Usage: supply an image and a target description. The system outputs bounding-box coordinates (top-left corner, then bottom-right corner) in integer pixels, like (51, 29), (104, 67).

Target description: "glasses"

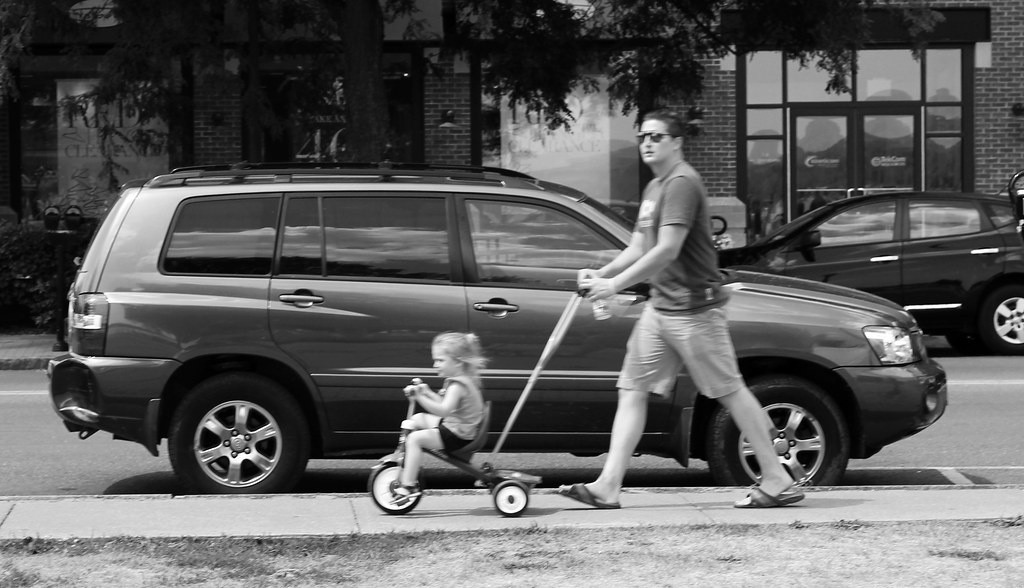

(636, 131), (680, 143)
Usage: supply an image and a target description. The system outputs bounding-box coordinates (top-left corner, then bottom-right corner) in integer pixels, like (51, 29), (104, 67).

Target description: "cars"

(722, 192), (1024, 357)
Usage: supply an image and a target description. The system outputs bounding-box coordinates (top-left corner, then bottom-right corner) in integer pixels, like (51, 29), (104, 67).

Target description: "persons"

(388, 331), (486, 505)
(551, 111), (805, 509)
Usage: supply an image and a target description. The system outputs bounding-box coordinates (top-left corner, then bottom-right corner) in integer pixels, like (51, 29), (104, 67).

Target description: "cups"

(592, 298), (613, 319)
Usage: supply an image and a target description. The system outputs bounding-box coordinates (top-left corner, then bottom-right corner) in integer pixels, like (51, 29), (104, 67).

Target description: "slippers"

(553, 482), (621, 509)
(734, 487), (805, 508)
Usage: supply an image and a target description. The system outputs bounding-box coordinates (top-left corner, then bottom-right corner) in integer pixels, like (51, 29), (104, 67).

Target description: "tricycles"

(368, 375), (544, 515)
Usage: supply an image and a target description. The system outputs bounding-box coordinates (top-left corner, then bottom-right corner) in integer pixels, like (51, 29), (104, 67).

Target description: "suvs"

(48, 162), (947, 496)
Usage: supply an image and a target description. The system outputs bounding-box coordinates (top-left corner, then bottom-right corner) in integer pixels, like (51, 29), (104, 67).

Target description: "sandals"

(389, 481), (422, 505)
